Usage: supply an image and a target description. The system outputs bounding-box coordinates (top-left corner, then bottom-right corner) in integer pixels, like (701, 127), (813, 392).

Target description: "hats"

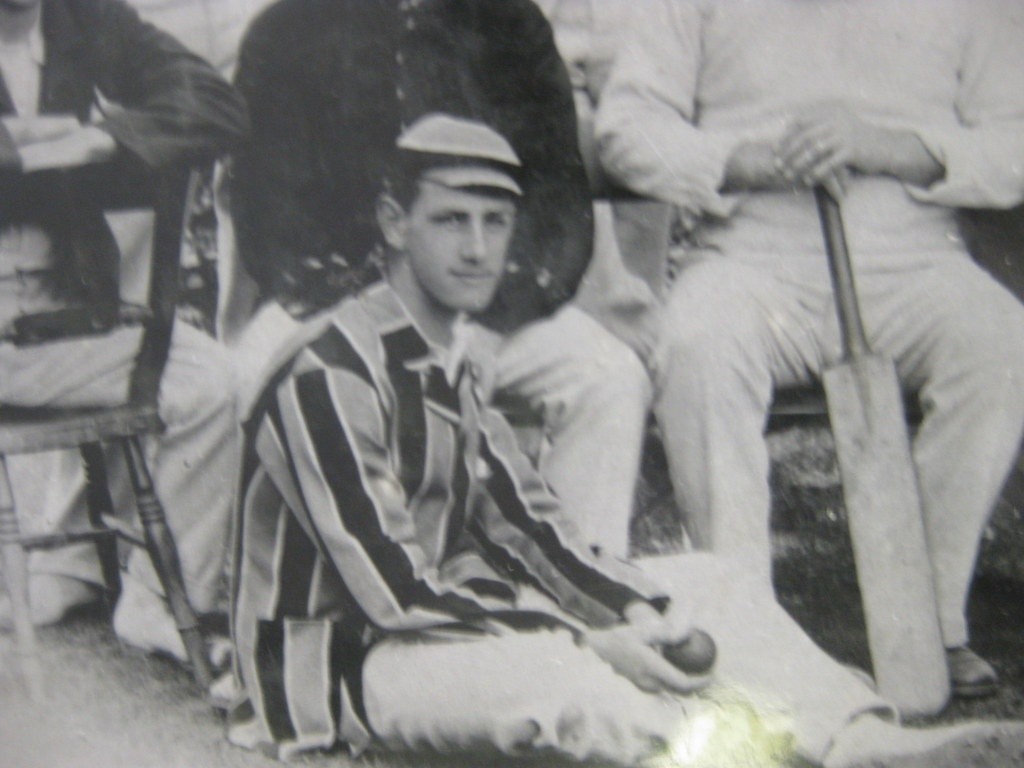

(396, 110), (523, 201)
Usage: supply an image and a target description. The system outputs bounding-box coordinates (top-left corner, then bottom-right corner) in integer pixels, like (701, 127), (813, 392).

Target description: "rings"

(817, 142), (828, 154)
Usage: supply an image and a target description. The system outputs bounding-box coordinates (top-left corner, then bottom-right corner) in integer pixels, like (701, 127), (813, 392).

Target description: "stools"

(0, 395), (208, 721)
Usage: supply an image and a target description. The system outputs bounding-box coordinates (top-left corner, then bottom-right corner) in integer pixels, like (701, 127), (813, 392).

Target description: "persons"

(0, 0), (251, 680)
(592, 0), (1024, 694)
(218, 0), (658, 557)
(225, 114), (1023, 768)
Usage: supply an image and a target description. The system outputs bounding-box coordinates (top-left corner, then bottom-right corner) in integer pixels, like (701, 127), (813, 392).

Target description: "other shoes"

(945, 645), (1002, 696)
(111, 586), (235, 670)
(823, 717), (1024, 768)
